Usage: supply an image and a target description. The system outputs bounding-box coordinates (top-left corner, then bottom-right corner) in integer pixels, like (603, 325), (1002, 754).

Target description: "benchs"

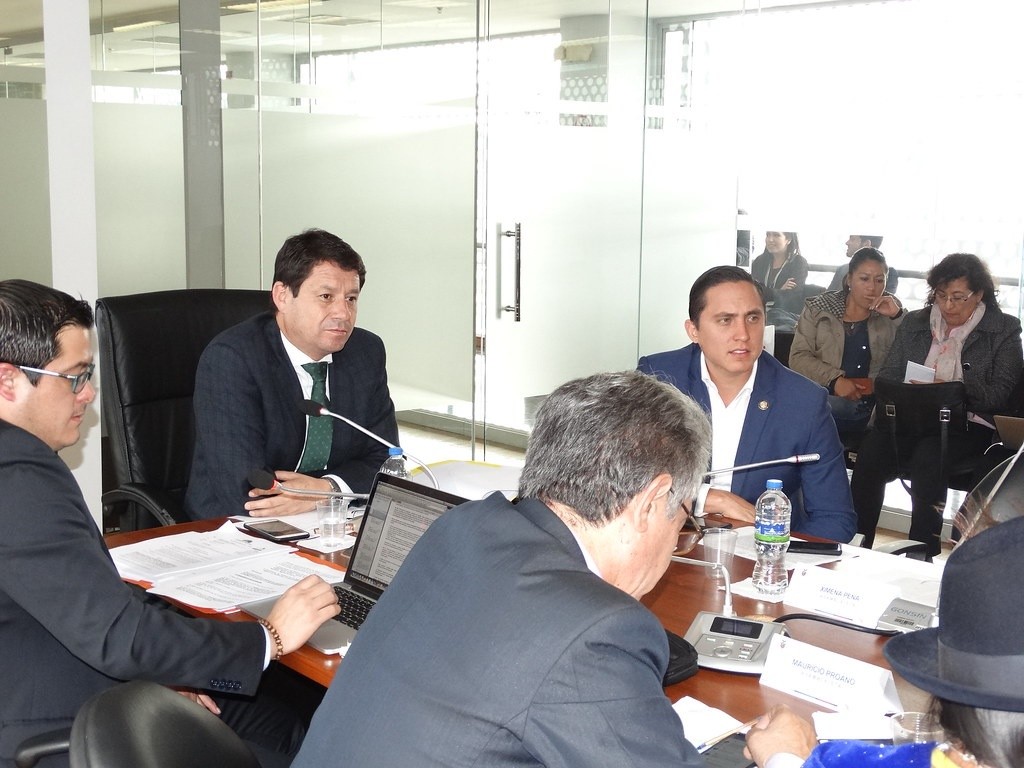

(773, 331), (1024, 491)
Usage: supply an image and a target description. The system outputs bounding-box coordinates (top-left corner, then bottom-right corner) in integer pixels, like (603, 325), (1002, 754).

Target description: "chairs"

(69, 681), (259, 768)
(798, 283), (828, 312)
(869, 445), (1023, 563)
(95, 288), (275, 536)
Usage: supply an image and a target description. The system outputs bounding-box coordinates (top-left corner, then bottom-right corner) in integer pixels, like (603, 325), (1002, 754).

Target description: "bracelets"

(257, 618), (284, 660)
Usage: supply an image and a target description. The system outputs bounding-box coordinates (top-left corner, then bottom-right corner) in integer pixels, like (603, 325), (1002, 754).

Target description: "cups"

(702, 527), (738, 580)
(315, 498), (350, 547)
(890, 711), (946, 746)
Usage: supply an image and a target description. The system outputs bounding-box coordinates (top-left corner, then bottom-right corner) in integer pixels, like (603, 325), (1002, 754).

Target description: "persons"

(850, 254), (1024, 563)
(787, 245), (909, 466)
(0, 278), (341, 768)
(180, 226), (406, 522)
(287, 364), (819, 767)
(826, 235), (897, 295)
(751, 231), (810, 308)
(635, 266), (859, 544)
(801, 515), (1024, 768)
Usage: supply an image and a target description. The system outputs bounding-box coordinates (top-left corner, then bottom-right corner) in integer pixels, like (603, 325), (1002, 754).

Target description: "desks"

(100, 512), (950, 768)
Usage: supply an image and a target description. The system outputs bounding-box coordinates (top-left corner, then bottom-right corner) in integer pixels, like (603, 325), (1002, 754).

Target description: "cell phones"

(698, 731), (758, 768)
(244, 519), (310, 541)
(683, 516), (733, 532)
(787, 541), (842, 556)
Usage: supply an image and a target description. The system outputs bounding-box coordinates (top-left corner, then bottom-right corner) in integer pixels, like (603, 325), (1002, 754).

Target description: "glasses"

(15, 364), (95, 395)
(933, 288), (974, 305)
(668, 488), (705, 555)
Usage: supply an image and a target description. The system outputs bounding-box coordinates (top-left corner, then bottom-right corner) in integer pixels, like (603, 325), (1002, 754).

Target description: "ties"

(296, 362), (333, 474)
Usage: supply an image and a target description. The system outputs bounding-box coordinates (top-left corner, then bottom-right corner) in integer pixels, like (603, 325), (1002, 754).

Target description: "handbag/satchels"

(875, 374), (966, 503)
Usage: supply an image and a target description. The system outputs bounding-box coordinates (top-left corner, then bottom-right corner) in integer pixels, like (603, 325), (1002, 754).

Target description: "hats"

(882, 515), (1024, 713)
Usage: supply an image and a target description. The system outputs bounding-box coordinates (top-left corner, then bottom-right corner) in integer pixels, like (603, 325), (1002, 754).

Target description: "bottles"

(752, 479), (792, 596)
(380, 447), (411, 483)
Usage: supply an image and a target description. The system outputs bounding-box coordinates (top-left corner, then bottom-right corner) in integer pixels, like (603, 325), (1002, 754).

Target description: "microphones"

(706, 453), (821, 476)
(296, 400), (439, 490)
(247, 470), (369, 499)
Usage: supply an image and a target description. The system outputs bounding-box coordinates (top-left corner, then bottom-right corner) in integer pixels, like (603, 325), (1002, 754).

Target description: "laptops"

(236, 472), (472, 654)
(993, 415), (1024, 450)
